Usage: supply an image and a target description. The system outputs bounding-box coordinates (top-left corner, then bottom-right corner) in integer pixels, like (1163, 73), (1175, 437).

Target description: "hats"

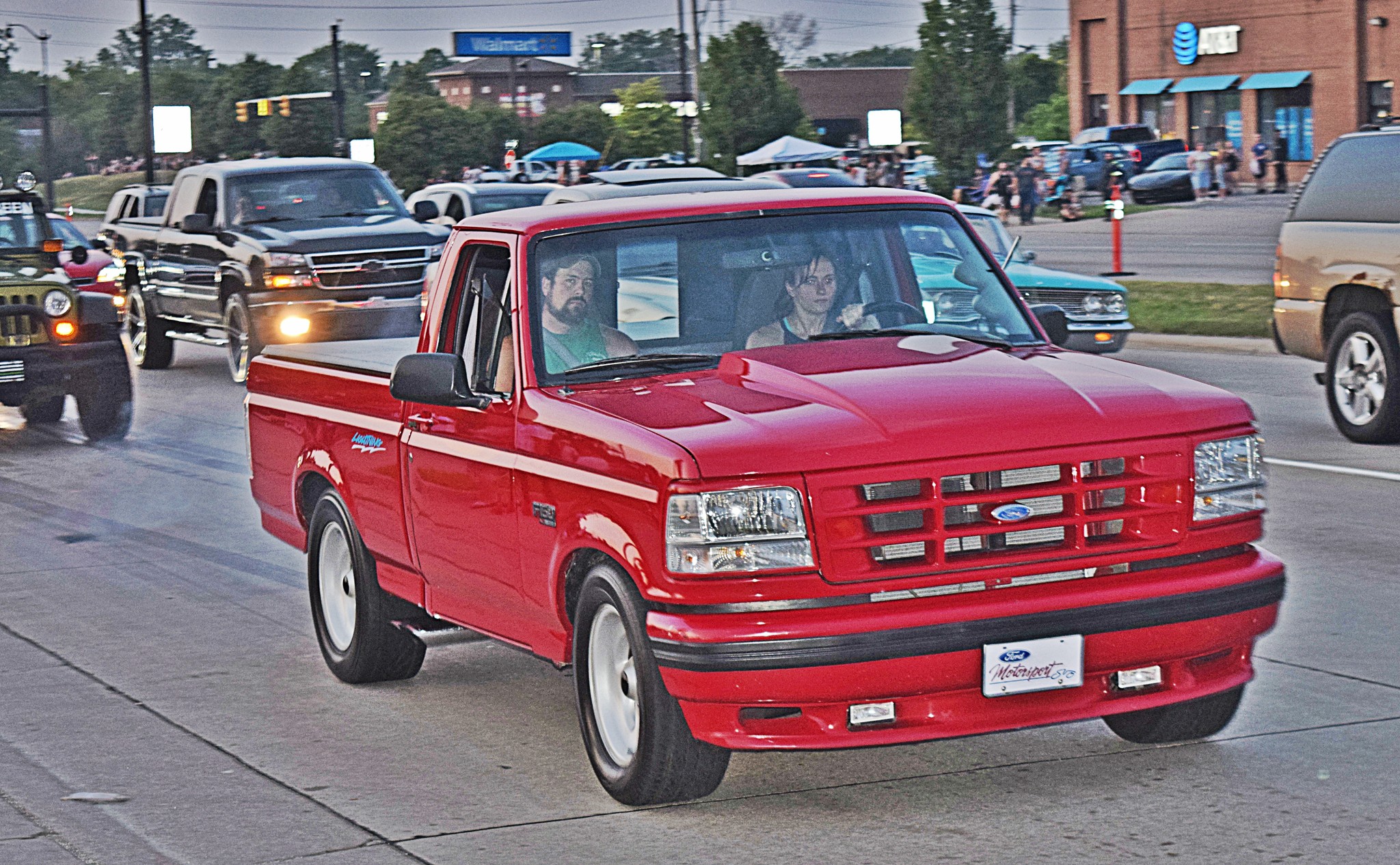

(539, 253), (602, 278)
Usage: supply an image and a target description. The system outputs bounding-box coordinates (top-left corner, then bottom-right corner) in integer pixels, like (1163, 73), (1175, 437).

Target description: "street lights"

(666, 34), (698, 166)
(2, 21), (55, 212)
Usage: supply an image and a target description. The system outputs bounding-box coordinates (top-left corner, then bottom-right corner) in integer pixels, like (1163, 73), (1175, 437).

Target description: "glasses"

(236, 201), (253, 206)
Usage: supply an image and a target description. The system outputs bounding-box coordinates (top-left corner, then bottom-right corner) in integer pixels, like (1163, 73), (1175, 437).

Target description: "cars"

(280, 158), (1134, 352)
(1128, 152), (1215, 205)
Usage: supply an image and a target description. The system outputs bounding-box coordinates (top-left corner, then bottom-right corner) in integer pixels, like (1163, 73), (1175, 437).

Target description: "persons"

(953, 145), (1084, 225)
(232, 191), (262, 225)
(1270, 129), (1290, 193)
(1250, 130), (1269, 194)
(1100, 152), (1123, 222)
(745, 246), (880, 352)
(849, 150), (932, 192)
(494, 253), (638, 396)
(1187, 140), (1240, 202)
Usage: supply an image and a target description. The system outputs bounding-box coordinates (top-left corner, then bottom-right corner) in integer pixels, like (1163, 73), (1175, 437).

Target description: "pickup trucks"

(241, 189), (1288, 809)
(1073, 126), (1186, 176)
(100, 156), (453, 385)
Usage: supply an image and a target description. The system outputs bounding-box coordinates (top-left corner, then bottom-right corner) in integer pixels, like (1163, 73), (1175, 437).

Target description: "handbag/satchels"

(1249, 158), (1261, 176)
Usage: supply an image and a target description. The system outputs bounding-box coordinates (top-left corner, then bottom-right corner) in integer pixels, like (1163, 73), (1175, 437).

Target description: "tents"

(735, 135), (844, 176)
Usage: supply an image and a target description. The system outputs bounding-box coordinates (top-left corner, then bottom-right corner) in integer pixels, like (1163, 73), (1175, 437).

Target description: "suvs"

(1036, 143), (1132, 191)
(1271, 114), (1400, 445)
(0, 172), (216, 444)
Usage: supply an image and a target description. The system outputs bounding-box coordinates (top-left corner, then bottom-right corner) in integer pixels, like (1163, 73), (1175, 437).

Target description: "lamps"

(1368, 18), (1385, 26)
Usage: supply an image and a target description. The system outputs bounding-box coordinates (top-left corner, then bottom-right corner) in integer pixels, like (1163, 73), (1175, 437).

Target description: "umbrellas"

(522, 142), (601, 161)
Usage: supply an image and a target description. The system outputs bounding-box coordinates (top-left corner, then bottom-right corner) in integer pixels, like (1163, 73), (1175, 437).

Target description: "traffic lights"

(236, 101), (247, 123)
(277, 97), (291, 118)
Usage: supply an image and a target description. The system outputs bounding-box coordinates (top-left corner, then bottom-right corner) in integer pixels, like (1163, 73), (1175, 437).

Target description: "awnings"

(1236, 71), (1313, 90)
(1118, 78), (1174, 95)
(1168, 75), (1237, 94)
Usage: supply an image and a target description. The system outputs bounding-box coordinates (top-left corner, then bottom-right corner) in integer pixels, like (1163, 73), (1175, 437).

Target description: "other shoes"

(1270, 190), (1286, 195)
(1195, 196), (1211, 202)
(1254, 188), (1267, 195)
